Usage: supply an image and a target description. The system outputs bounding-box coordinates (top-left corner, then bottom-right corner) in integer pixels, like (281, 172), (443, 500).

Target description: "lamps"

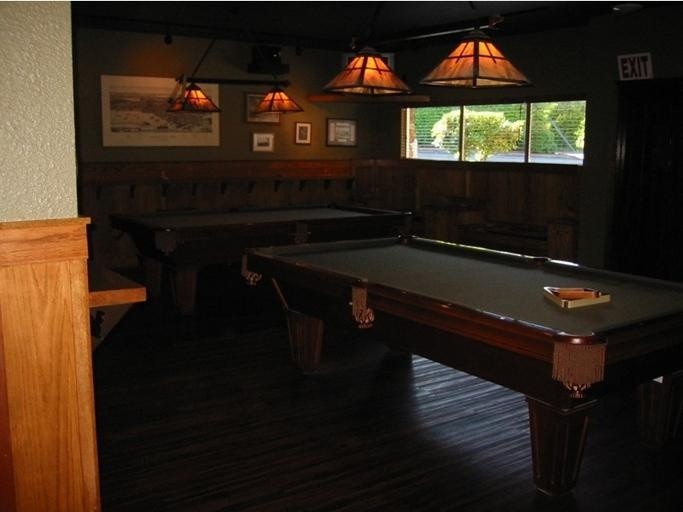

(321, 2), (415, 97)
(256, 72), (304, 114)
(418, 1), (533, 89)
(166, 37), (221, 112)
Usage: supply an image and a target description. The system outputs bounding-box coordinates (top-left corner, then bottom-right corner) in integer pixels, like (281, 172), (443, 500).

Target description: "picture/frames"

(244, 91), (281, 124)
(295, 122), (312, 145)
(100, 75), (221, 148)
(252, 133), (276, 154)
(326, 117), (359, 148)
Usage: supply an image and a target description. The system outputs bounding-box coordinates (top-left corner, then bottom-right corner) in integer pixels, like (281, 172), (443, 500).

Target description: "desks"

(108, 203), (414, 315)
(242, 233), (683, 498)
